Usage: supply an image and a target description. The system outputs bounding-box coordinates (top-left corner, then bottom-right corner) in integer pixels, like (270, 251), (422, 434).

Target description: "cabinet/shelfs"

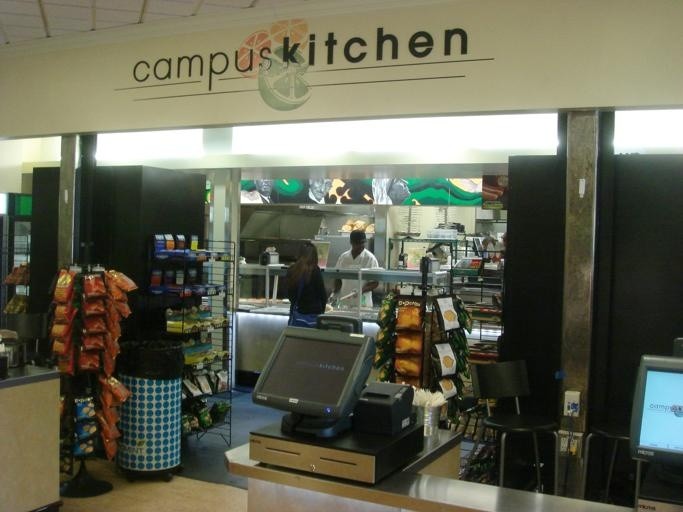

(421, 238), (504, 443)
(0, 217), (30, 313)
(150, 232), (234, 450)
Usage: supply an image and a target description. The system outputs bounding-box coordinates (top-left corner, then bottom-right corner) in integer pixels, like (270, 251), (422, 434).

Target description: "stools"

(583, 405), (641, 508)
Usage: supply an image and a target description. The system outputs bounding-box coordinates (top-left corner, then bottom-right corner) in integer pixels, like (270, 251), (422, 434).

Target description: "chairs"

(470, 360), (561, 494)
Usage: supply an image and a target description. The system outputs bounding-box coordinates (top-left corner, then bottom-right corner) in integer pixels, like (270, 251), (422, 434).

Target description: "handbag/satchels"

(288, 311), (317, 328)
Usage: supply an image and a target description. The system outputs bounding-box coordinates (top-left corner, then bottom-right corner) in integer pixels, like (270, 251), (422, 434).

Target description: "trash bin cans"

(118, 348), (184, 481)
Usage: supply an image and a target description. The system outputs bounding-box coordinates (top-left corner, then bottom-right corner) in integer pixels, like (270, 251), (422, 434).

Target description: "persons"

(284, 241), (328, 329)
(328, 229), (381, 327)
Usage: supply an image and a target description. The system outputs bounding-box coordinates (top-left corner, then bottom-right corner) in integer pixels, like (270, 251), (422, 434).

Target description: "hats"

(350, 230), (367, 243)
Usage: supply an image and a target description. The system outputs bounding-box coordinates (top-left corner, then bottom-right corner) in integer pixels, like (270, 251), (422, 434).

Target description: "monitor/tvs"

(252, 326), (376, 419)
(630, 354), (683, 466)
